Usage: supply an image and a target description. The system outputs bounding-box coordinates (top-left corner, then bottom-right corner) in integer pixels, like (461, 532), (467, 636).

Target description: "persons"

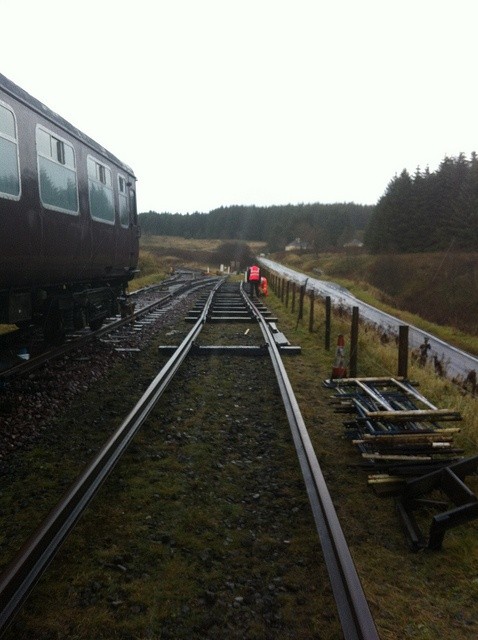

(246, 261), (261, 296)
(257, 277), (269, 296)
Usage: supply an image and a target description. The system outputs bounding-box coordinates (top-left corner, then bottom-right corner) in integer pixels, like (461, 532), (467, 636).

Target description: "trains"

(0, 74), (140, 350)
